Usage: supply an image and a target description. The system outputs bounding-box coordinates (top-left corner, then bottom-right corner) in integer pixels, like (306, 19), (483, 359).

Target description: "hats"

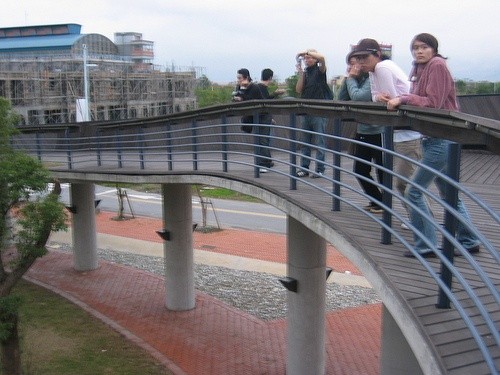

(351, 39), (383, 56)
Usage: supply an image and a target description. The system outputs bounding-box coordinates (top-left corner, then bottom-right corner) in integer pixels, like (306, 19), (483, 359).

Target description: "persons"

(352, 38), (435, 229)
(295, 49), (333, 178)
(234, 68), (285, 173)
(336, 50), (386, 213)
(387, 33), (483, 258)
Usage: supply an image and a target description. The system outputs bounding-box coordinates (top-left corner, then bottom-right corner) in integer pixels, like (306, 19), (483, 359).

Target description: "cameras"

(297, 59), (301, 64)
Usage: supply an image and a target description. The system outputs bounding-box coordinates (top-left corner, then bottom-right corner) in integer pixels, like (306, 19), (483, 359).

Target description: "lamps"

(193, 223), (197, 231)
(326, 268), (331, 278)
(95, 200), (101, 207)
(65, 207), (76, 213)
(156, 230), (170, 241)
(279, 277), (296, 294)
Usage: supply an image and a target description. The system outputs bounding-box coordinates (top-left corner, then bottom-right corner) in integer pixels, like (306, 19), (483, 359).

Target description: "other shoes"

(260, 167), (269, 173)
(363, 204), (384, 213)
(311, 172), (324, 178)
(403, 250), (437, 259)
(455, 246), (480, 256)
(401, 222), (412, 230)
(295, 170), (309, 177)
